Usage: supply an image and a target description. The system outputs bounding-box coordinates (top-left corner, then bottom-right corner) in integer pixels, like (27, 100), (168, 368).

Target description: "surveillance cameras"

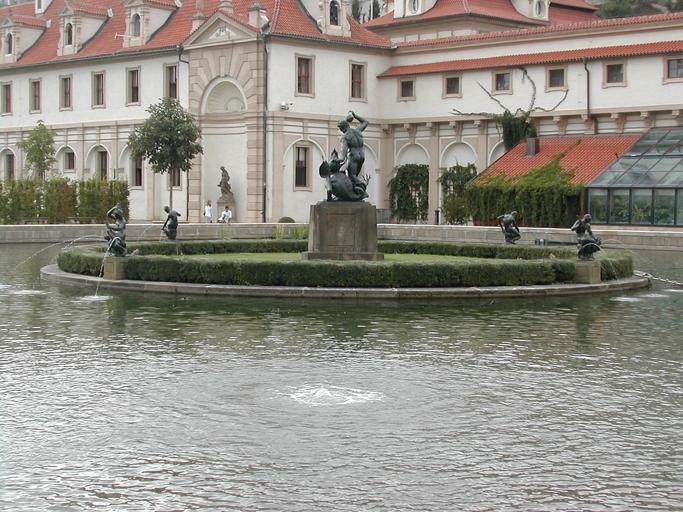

(280, 101), (287, 111)
(289, 100), (296, 108)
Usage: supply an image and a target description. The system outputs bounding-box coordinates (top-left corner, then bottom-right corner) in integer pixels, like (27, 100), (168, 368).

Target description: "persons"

(220, 166), (230, 195)
(205, 200), (212, 223)
(161, 206), (181, 239)
(496, 210), (521, 243)
(571, 214), (601, 249)
(220, 205), (232, 223)
(104, 203), (127, 257)
(337, 110), (367, 193)
